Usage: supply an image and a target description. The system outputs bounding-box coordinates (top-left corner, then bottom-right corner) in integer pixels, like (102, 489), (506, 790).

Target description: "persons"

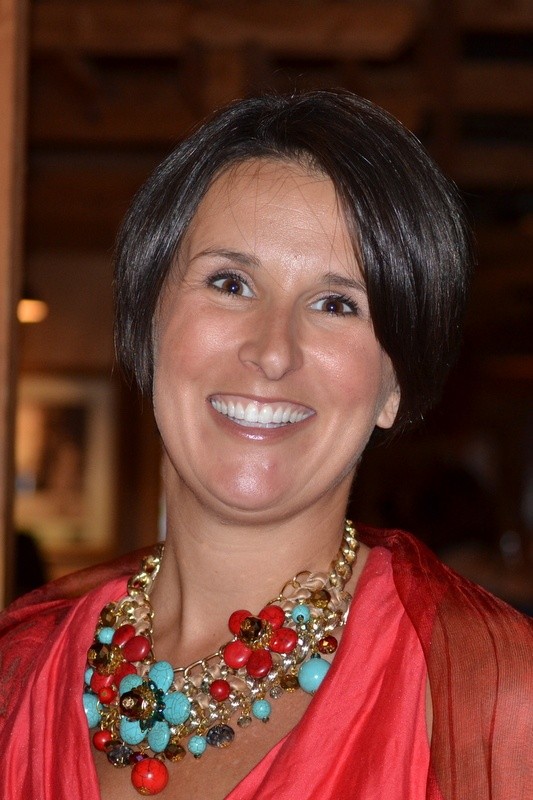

(0, 87), (531, 798)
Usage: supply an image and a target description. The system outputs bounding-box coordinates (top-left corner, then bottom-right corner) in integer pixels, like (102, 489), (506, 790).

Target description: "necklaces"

(82, 516), (364, 795)
